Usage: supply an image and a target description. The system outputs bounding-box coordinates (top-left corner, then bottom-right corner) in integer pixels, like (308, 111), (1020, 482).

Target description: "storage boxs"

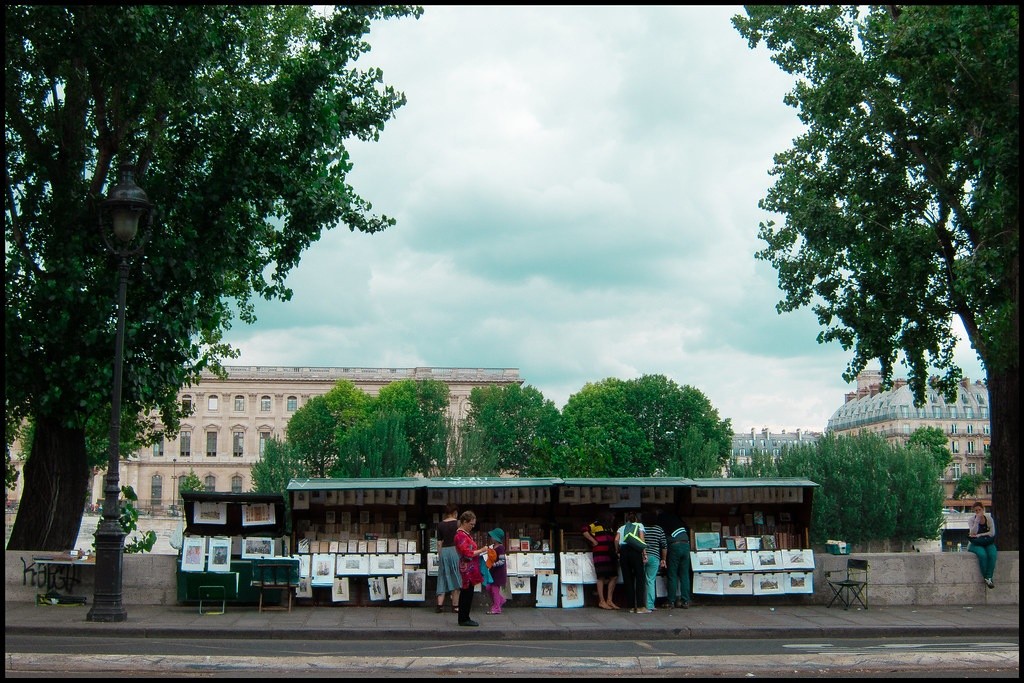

(824, 541), (851, 555)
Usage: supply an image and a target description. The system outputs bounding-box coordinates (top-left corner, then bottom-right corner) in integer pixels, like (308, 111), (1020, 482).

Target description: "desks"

(33, 559), (96, 606)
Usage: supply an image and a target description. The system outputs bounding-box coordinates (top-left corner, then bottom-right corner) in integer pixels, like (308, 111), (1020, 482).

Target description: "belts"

(670, 540), (687, 544)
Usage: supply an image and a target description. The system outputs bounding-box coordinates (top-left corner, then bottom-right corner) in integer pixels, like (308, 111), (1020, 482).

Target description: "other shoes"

(435, 605), (444, 613)
(486, 610), (500, 615)
(606, 602), (620, 610)
(459, 620), (479, 626)
(629, 608), (637, 613)
(500, 599), (506, 606)
(599, 602), (613, 609)
(452, 606), (458, 613)
(662, 601), (675, 608)
(675, 601), (689, 609)
(636, 607), (652, 614)
(984, 578), (995, 589)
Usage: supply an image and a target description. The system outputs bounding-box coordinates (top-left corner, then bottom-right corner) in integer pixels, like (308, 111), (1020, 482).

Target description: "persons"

(615, 512), (652, 614)
(454, 511), (494, 626)
(485, 528), (507, 614)
(967, 502), (997, 588)
(581, 510), (620, 610)
(656, 509), (691, 609)
(641, 514), (668, 611)
(957, 542), (962, 552)
(436, 503), (462, 613)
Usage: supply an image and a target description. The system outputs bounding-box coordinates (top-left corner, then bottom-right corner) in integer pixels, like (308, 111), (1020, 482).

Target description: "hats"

(488, 528), (504, 543)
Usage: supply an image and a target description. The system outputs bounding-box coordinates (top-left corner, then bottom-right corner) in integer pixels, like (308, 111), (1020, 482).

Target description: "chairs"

(257, 563), (296, 613)
(826, 559), (869, 610)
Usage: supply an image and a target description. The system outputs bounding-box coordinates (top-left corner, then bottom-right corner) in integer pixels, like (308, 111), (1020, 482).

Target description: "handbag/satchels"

(968, 536), (993, 546)
(623, 523), (648, 552)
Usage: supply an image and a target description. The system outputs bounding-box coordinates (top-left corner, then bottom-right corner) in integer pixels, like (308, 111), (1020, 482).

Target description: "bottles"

(283, 545), (288, 556)
(957, 541), (961, 552)
(78, 548), (82, 558)
